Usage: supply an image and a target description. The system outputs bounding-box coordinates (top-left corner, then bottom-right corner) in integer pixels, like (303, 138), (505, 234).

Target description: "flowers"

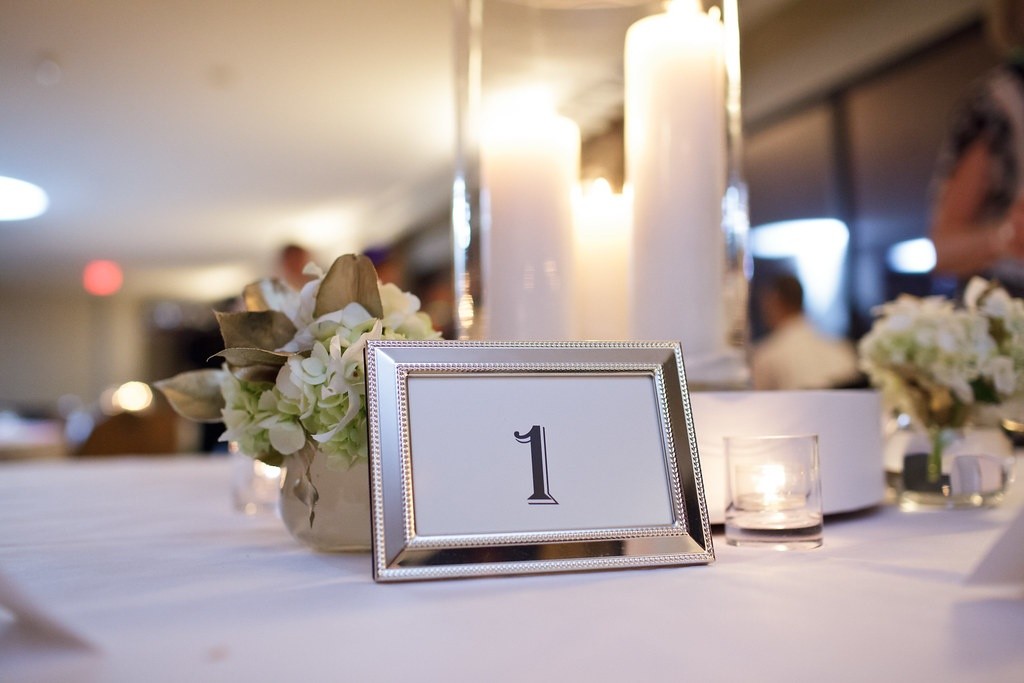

(151, 252), (447, 471)
(856, 273), (1024, 490)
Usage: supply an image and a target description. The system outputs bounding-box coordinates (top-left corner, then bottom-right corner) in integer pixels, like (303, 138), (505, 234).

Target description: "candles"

(622, 1), (754, 387)
(475, 76), (586, 347)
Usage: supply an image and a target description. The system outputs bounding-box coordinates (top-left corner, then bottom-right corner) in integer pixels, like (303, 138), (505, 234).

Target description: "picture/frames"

(363, 337), (718, 586)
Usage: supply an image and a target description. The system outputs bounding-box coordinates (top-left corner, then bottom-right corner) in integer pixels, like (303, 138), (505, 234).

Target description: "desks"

(0, 447), (1022, 683)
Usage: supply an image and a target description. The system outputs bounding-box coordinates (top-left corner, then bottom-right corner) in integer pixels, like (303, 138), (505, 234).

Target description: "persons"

(927, 56), (1024, 301)
(747, 275), (856, 391)
(278, 240), (457, 344)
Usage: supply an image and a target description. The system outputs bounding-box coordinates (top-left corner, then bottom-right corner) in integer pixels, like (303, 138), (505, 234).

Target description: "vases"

(877, 414), (1017, 513)
(275, 436), (372, 554)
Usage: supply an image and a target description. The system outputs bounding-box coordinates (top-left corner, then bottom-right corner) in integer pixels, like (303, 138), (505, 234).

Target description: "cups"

(721, 434), (823, 552)
